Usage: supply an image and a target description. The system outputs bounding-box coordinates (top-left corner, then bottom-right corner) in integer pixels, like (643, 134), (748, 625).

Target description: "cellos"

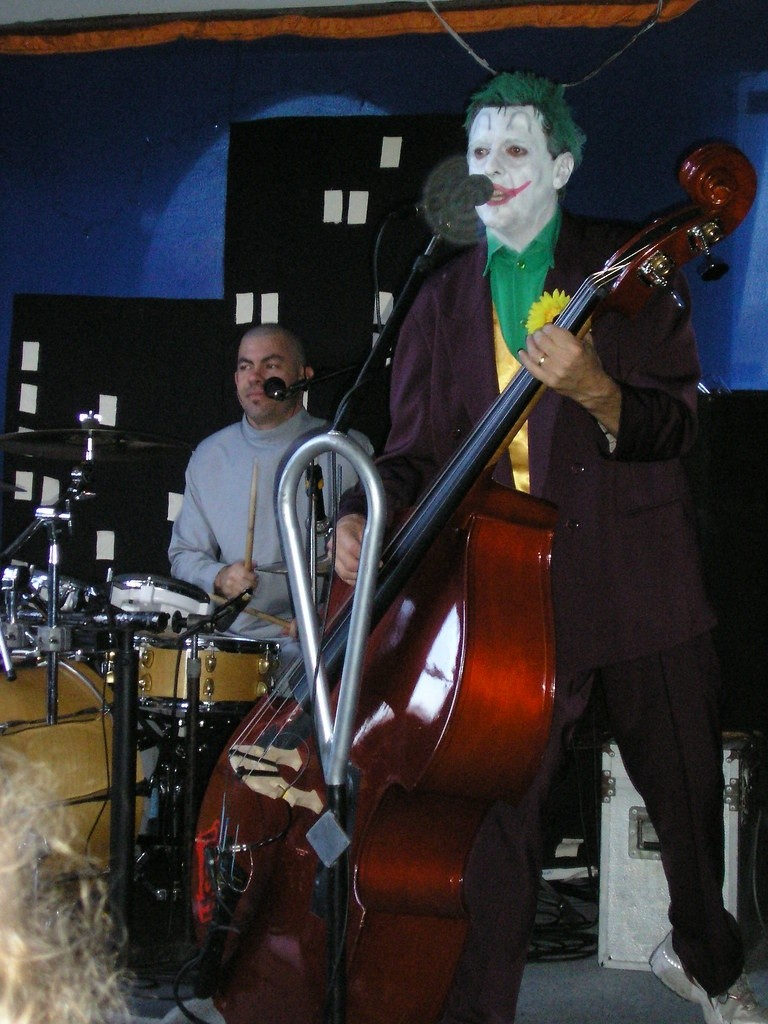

(191, 139), (759, 1022)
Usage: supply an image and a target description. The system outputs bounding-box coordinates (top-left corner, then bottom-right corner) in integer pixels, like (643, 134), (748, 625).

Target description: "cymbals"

(0, 410), (196, 472)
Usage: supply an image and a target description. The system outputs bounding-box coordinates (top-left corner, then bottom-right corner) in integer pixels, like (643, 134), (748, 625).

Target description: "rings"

(538, 352), (547, 367)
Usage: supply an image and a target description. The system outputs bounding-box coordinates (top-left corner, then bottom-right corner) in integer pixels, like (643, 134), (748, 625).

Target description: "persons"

(0, 324), (375, 1024)
(326, 71), (768, 1024)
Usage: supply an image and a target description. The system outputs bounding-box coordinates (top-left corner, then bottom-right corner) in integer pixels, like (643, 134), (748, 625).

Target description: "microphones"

(192, 861), (249, 999)
(388, 174), (493, 224)
(213, 586), (256, 634)
(264, 376), (287, 401)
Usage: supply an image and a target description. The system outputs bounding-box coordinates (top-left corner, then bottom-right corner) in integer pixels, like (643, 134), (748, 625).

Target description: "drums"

(106, 573), (212, 640)
(136, 631), (282, 718)
(1, 654), (151, 913)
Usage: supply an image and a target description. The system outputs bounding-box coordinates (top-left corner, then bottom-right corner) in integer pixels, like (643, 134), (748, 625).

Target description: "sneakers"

(650, 928), (768, 1024)
